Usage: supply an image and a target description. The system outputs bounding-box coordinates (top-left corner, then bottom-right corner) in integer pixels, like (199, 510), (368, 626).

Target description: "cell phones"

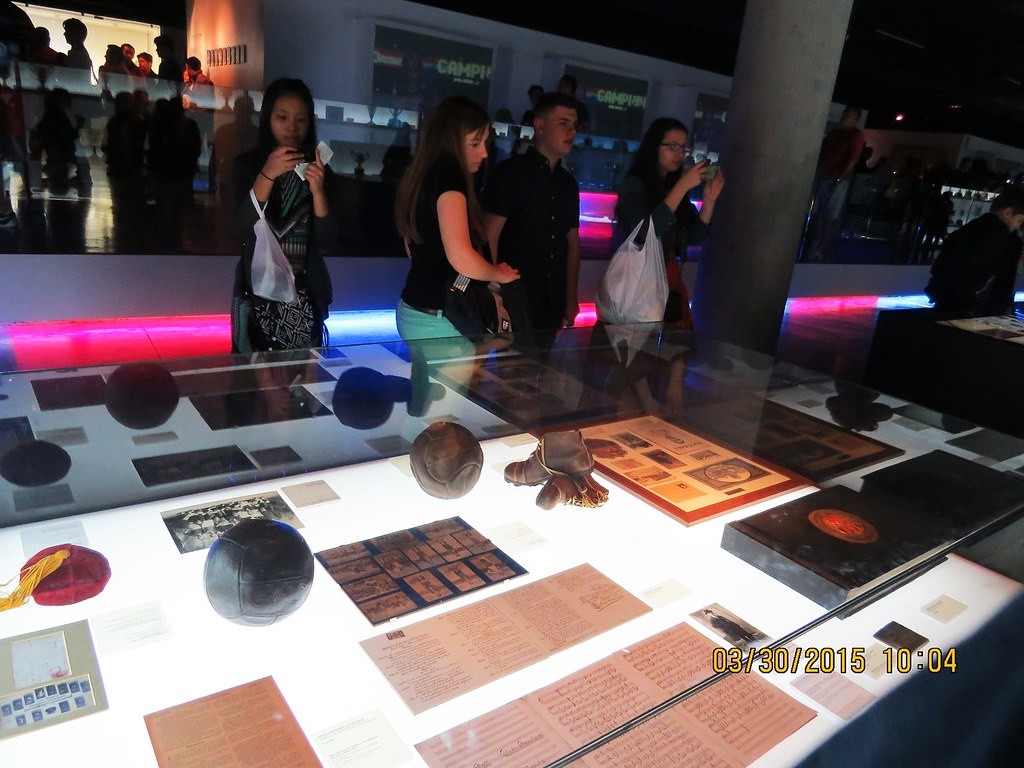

(704, 166), (718, 180)
(287, 148), (316, 163)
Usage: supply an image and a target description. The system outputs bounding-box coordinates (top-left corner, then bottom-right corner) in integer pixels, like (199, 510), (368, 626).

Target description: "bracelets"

(260, 170), (277, 182)
(259, 378), (274, 387)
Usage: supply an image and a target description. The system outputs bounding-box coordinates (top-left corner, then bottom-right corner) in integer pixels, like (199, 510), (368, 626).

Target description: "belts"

(415, 305), (437, 315)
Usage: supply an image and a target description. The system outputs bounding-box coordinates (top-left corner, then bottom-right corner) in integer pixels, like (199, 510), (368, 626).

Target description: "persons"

(394, 96), (519, 340)
(809, 100), (863, 250)
(855, 146), (1024, 232)
(230, 78), (337, 354)
(233, 349), (321, 422)
(610, 116), (725, 328)
(480, 91), (581, 329)
(20, 18), (730, 258)
(923, 187), (1024, 317)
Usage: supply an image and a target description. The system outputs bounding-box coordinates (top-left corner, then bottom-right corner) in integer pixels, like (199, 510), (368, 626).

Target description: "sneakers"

(505, 429), (593, 485)
(536, 470), (609, 510)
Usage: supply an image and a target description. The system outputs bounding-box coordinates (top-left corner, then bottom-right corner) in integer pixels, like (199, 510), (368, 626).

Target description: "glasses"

(659, 141), (689, 154)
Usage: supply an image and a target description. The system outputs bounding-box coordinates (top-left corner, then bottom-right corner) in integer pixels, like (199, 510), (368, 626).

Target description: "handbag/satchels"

(595, 216), (669, 324)
(476, 292), (514, 353)
(248, 188), (297, 303)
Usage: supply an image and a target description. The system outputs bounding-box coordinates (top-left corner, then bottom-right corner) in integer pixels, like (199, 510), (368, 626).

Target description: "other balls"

(104, 363), (181, 431)
(409, 423), (481, 498)
(205, 519), (314, 624)
